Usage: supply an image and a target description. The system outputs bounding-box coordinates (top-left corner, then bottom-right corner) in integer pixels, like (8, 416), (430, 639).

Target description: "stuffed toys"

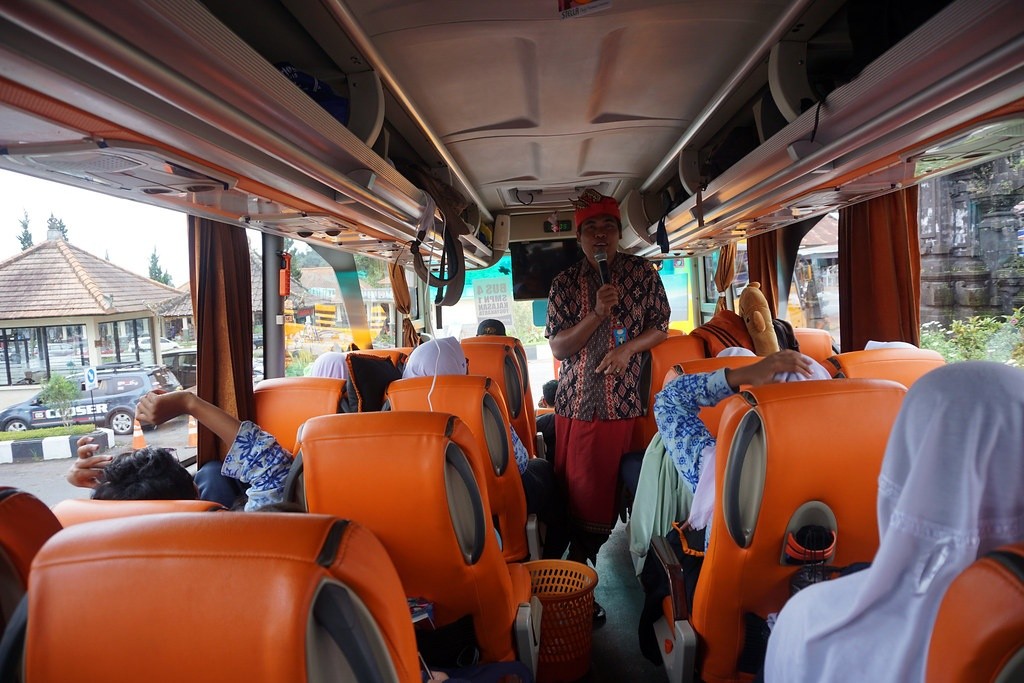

(740, 281), (780, 356)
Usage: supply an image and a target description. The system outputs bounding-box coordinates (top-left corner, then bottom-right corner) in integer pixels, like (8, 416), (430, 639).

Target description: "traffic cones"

(130, 420), (152, 451)
(184, 415), (197, 449)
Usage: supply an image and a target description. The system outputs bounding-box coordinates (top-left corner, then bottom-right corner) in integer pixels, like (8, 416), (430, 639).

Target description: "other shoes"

(591, 601), (606, 627)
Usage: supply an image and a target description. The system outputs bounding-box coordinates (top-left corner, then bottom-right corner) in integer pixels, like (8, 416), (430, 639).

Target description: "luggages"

(710, 125), (758, 180)
(847, 0), (950, 68)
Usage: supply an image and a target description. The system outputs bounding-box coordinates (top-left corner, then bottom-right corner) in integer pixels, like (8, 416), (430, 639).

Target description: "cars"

(128, 336), (179, 354)
(78, 345), (124, 357)
(162, 347), (197, 390)
(253, 336), (263, 350)
(45, 342), (75, 357)
(0, 343), (40, 362)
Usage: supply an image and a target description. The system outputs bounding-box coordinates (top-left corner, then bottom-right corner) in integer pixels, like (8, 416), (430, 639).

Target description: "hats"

(568, 189), (621, 230)
(478, 319), (506, 335)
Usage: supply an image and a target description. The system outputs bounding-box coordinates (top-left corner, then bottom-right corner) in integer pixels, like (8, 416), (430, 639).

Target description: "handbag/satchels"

(390, 156), (467, 306)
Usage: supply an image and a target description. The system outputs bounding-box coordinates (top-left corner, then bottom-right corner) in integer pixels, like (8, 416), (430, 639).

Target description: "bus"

(280, 298), (387, 362)
(648, 248), (814, 339)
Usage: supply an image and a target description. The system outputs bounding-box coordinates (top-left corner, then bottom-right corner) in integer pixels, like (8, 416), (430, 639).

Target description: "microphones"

(594, 249), (619, 317)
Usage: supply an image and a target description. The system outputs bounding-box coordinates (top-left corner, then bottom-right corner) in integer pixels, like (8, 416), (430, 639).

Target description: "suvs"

(0, 361), (184, 435)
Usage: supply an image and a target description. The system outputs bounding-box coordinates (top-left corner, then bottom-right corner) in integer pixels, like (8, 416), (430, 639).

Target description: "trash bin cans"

(522, 559), (599, 683)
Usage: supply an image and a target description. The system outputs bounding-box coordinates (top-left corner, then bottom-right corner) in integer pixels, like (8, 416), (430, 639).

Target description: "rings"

(615, 368), (620, 373)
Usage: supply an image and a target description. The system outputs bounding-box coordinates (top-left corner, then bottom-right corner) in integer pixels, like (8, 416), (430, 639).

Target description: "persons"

(626, 346), (757, 580)
(764, 360), (1024, 683)
(306, 318), (560, 505)
(67, 389), (296, 513)
(546, 187), (672, 624)
(653, 348), (833, 585)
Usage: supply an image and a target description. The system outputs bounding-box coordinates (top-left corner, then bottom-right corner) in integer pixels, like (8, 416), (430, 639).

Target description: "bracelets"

(593, 308), (603, 323)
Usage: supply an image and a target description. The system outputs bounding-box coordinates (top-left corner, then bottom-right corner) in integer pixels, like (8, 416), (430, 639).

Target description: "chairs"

(0, 332), (1024, 683)
